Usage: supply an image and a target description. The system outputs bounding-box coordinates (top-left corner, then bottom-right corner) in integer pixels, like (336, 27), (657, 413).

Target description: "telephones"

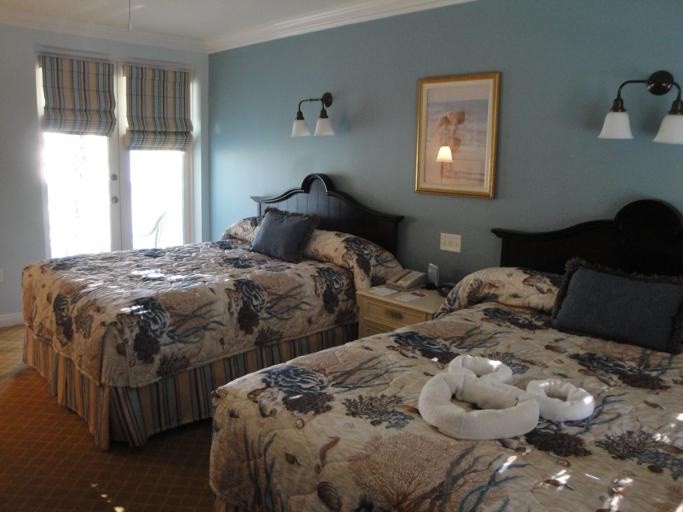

(386, 268), (426, 291)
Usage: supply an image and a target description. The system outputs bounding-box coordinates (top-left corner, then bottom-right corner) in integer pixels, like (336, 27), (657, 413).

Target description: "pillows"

(249, 207), (320, 264)
(550, 258), (683, 353)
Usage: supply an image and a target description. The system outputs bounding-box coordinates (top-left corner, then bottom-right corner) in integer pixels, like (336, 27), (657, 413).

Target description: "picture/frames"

(415, 72), (501, 198)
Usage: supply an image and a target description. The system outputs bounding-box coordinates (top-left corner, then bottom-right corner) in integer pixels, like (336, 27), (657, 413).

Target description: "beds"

(22, 174), (404, 451)
(209, 200), (683, 512)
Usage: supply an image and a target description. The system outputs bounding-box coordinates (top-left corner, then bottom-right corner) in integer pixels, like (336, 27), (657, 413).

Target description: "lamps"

(435, 101), (454, 163)
(597, 70), (683, 145)
(291, 92), (335, 137)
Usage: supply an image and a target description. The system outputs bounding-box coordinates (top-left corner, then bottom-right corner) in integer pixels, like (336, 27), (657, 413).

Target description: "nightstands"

(355, 282), (447, 339)
